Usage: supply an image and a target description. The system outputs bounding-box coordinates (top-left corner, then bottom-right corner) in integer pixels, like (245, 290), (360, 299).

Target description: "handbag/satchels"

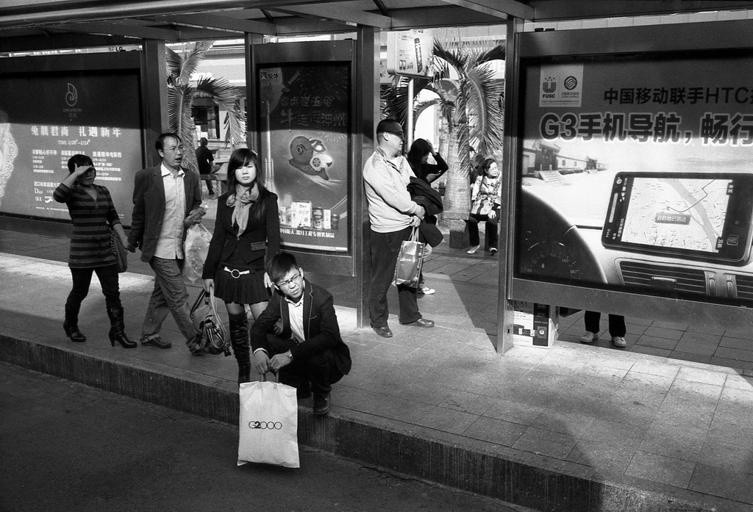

(391, 240), (426, 290)
(187, 303), (231, 355)
(233, 379), (301, 471)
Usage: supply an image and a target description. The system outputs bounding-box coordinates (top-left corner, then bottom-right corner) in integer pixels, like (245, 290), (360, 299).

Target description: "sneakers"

(489, 247), (498, 253)
(466, 244), (481, 254)
(141, 331), (172, 349)
(418, 283), (436, 296)
(294, 383), (312, 401)
(312, 390), (332, 416)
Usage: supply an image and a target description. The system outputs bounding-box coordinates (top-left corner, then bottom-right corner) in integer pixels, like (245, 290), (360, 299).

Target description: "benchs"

(440, 210), (501, 252)
(201, 172), (228, 198)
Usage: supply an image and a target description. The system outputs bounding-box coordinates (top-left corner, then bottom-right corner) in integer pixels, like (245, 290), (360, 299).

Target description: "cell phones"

(434, 170), (441, 174)
(601, 172), (753, 266)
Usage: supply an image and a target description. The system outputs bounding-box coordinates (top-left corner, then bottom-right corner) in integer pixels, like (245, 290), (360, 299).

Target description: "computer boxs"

(533, 303), (549, 346)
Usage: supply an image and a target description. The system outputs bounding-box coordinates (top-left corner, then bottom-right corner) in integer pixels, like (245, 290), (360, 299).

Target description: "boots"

(62, 302), (86, 343)
(227, 309), (251, 387)
(106, 306), (137, 349)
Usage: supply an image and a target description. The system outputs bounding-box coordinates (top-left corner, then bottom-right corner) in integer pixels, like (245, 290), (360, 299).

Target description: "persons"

(193, 137), (221, 196)
(202, 148), (279, 389)
(51, 154), (135, 348)
(409, 137), (447, 296)
(464, 158), (502, 256)
(580, 309), (626, 347)
(247, 252), (351, 415)
(361, 118), (436, 337)
(128, 133), (210, 355)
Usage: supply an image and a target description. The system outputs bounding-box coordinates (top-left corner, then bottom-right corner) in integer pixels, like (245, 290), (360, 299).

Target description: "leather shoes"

(374, 324), (392, 338)
(402, 316), (434, 329)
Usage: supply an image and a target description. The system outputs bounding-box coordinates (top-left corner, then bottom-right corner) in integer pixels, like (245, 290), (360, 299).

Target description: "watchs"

(284, 351), (294, 358)
